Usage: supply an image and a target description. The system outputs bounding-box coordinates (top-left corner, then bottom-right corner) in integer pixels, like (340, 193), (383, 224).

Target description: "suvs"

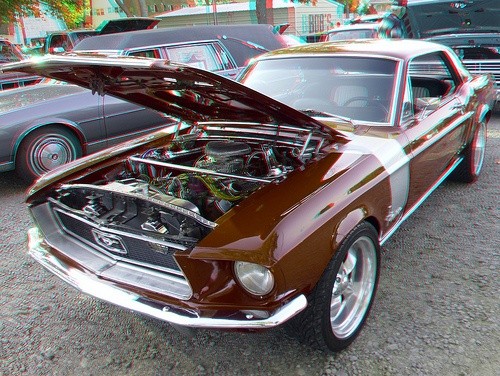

(0, 25), (289, 187)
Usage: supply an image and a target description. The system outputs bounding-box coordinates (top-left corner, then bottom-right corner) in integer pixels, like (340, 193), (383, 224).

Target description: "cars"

(0, 37), (499, 352)
(319, 1), (500, 104)
(1, 16), (162, 65)
